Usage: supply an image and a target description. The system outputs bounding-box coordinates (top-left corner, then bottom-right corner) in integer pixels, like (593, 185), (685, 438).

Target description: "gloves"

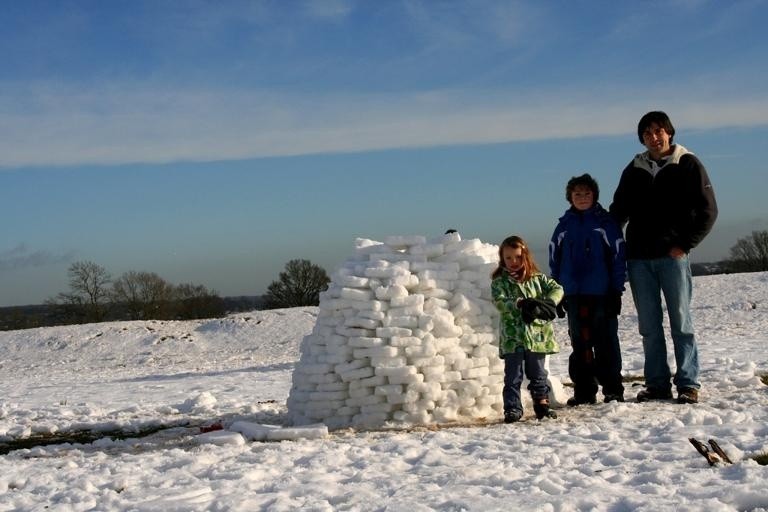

(517, 297), (556, 325)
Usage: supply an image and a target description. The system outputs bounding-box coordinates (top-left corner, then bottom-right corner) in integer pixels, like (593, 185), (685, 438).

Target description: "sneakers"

(504, 410), (523, 424)
(567, 395), (597, 407)
(636, 387), (673, 402)
(604, 392), (625, 403)
(534, 403), (557, 421)
(678, 387), (699, 404)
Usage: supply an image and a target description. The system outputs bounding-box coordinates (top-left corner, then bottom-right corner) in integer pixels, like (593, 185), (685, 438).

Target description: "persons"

(489, 234), (565, 424)
(547, 173), (628, 407)
(607, 109), (719, 403)
